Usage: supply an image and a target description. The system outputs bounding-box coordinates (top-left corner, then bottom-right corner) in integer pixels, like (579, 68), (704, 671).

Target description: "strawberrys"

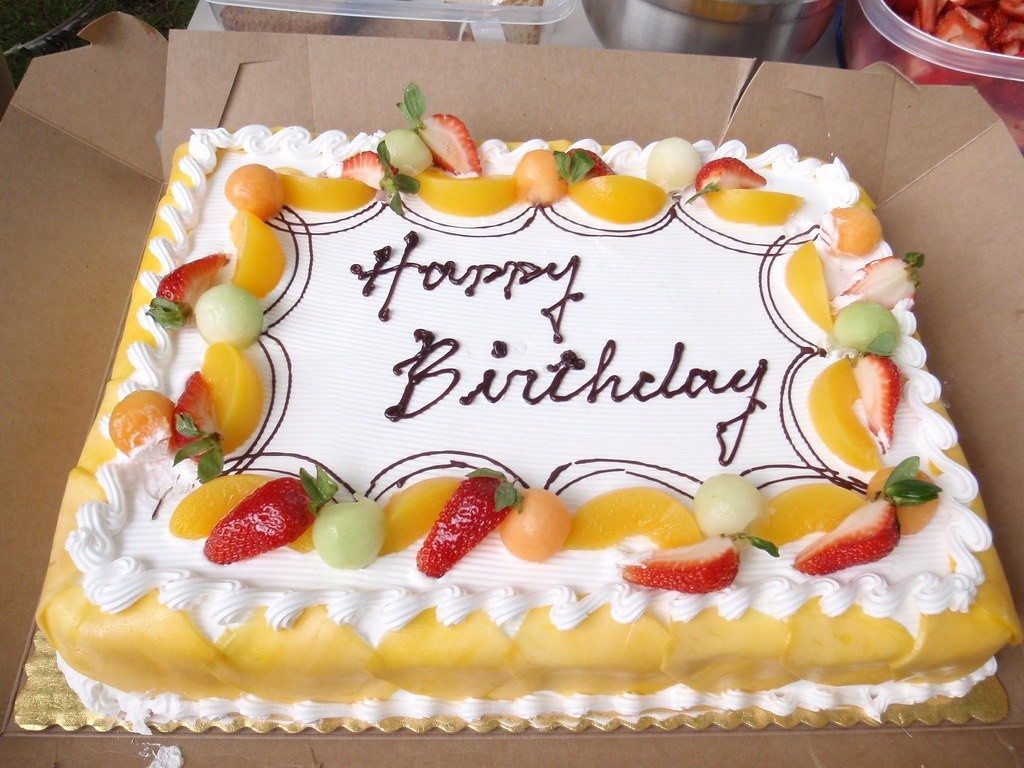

(839, 0), (1024, 148)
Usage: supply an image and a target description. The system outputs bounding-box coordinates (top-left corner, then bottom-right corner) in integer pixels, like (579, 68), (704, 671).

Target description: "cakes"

(32, 81), (1022, 728)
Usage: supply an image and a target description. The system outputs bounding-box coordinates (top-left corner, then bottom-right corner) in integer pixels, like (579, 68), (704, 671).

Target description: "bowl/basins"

(581, 0), (840, 64)
